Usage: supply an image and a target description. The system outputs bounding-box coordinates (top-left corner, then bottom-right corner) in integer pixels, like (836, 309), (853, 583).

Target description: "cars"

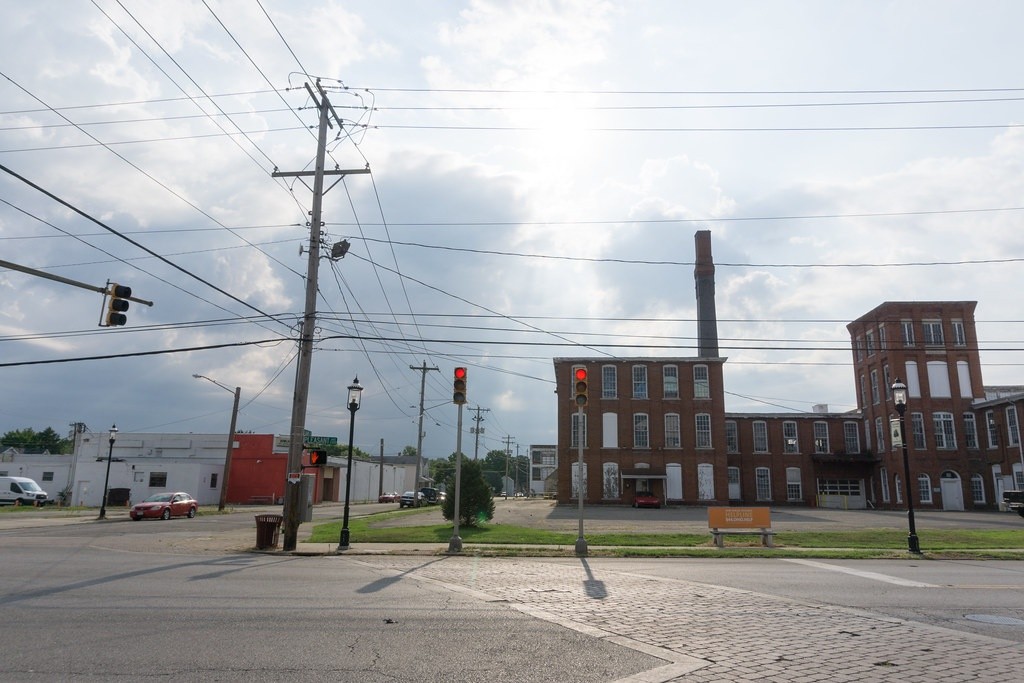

(379, 487), (448, 509)
(501, 492), (508, 497)
(631, 490), (662, 509)
(129, 492), (199, 520)
(514, 492), (524, 497)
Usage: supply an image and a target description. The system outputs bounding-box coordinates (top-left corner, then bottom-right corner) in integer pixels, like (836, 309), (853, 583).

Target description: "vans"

(0, 477), (48, 506)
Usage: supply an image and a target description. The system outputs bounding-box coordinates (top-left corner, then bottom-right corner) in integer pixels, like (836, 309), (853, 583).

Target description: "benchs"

(707, 507), (777, 547)
(249, 495), (273, 504)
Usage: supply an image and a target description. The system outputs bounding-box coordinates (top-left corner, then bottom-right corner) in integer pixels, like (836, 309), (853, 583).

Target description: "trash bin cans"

(254, 514), (282, 550)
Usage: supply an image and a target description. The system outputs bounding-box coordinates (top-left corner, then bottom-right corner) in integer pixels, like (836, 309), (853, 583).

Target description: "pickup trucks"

(1001, 490), (1024, 517)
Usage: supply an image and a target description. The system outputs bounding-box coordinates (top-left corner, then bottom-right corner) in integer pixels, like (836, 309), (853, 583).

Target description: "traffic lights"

(310, 451), (327, 467)
(106, 285), (132, 327)
(453, 366), (467, 405)
(572, 365), (589, 407)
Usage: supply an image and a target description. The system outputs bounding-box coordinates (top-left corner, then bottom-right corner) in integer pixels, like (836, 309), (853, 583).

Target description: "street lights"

(192, 374), (241, 512)
(338, 374), (364, 550)
(890, 376), (921, 552)
(97, 423), (119, 519)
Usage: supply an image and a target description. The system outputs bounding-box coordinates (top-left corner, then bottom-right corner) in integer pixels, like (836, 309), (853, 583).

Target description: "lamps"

(256, 460), (262, 464)
